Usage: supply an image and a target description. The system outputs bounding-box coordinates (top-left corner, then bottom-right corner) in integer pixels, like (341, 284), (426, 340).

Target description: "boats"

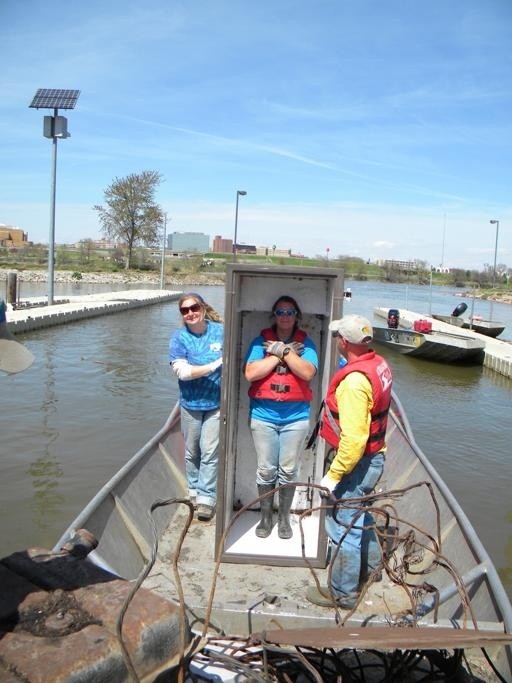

(1, 323), (511, 683)
(429, 312), (505, 338)
(361, 326), (486, 361)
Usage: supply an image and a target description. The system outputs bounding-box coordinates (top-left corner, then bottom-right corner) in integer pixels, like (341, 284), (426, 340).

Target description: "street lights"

(29, 87), (82, 306)
(489, 219), (499, 287)
(233, 188), (247, 262)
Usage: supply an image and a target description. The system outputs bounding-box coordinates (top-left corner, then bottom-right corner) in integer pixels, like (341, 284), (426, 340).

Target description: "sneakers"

(190, 496), (215, 520)
(361, 571), (382, 582)
(306, 586), (352, 610)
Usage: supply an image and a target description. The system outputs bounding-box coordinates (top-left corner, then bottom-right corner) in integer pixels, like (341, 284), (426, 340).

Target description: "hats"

(329, 315), (374, 345)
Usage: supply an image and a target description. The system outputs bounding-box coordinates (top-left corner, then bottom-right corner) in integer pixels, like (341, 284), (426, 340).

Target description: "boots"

(278, 482), (296, 538)
(255, 483), (275, 538)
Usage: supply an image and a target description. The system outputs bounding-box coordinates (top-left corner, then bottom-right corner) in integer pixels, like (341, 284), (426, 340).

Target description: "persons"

(242, 296), (320, 538)
(170, 292), (225, 520)
(303, 315), (393, 611)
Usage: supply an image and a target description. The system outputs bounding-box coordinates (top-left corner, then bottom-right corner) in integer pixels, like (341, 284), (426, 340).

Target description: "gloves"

(318, 475), (340, 499)
(263, 340), (305, 366)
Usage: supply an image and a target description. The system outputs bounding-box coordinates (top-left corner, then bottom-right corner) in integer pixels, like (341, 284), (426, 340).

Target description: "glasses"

(180, 303), (200, 315)
(274, 308), (298, 317)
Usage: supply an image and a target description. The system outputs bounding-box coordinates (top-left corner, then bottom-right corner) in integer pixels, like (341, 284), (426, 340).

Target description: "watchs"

(281, 347), (291, 359)
